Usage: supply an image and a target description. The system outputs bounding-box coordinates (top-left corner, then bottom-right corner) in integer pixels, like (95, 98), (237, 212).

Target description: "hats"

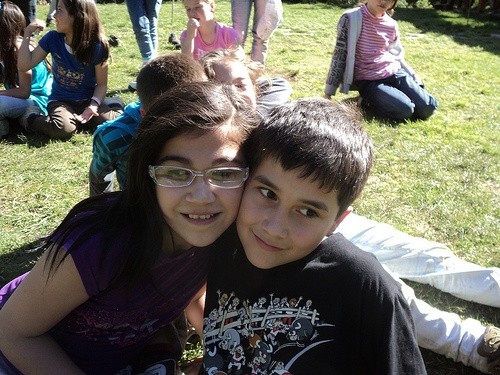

(256, 0), (282, 40)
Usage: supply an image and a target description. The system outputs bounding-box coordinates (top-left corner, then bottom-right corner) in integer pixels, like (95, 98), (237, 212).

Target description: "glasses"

(149, 165), (250, 189)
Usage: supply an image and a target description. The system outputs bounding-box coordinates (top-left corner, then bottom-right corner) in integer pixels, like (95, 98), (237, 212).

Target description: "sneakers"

(103, 98), (125, 108)
(21, 107), (40, 130)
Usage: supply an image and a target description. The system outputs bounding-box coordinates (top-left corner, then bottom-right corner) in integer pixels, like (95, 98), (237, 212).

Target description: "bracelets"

(91, 96), (101, 106)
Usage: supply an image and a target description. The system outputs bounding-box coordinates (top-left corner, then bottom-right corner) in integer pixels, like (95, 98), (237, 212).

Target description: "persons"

(0, 2), (54, 138)
(128, 0), (243, 93)
(125, 0), (162, 66)
(17, 0), (124, 140)
(89, 47), (500, 375)
(0, 0), (56, 37)
(181, 97), (427, 375)
(231, 0), (275, 65)
(0, 81), (264, 375)
(324, 0), (437, 122)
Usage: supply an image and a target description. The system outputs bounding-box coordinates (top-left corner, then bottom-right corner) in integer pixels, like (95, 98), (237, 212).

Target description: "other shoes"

(0, 119), (9, 135)
(128, 82), (137, 93)
(477, 325), (500, 375)
